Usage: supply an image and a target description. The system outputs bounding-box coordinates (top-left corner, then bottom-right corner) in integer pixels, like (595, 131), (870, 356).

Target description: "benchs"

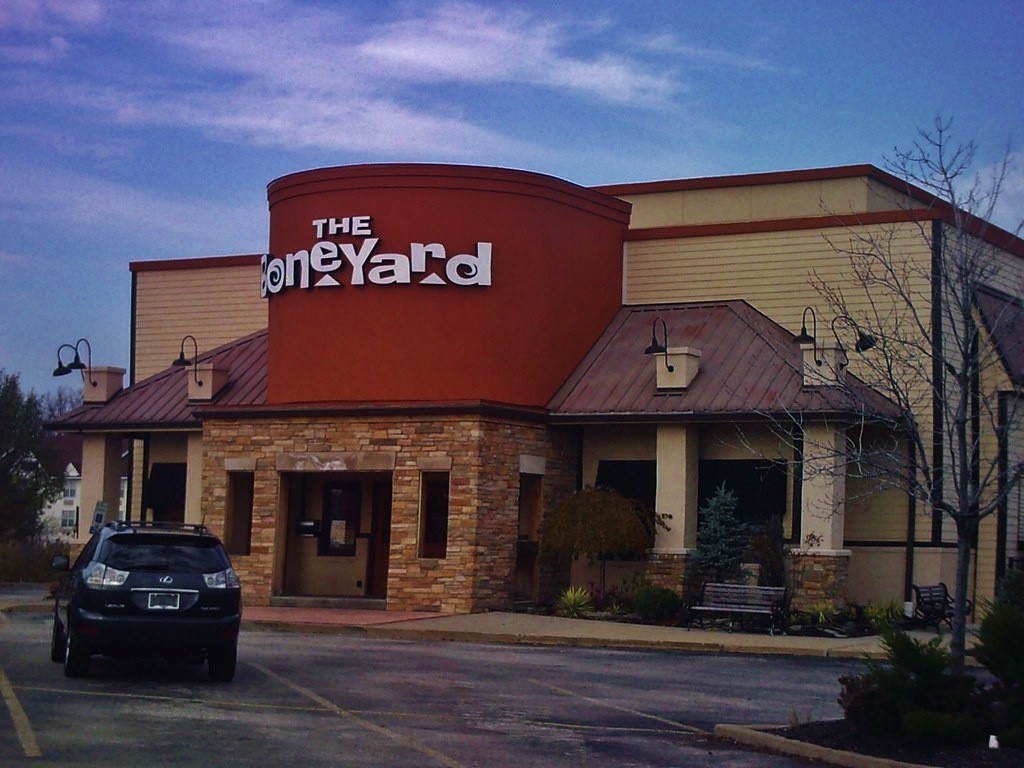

(904, 582), (971, 635)
(681, 580), (790, 636)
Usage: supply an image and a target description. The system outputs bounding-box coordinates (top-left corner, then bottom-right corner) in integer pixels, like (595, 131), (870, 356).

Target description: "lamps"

(643, 315), (674, 373)
(172, 335), (203, 387)
(67, 338), (98, 388)
(52, 343), (85, 383)
(792, 306), (822, 367)
(832, 315), (878, 371)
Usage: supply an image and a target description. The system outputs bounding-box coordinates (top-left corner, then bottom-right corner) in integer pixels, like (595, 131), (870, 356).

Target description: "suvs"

(50, 518), (242, 680)
